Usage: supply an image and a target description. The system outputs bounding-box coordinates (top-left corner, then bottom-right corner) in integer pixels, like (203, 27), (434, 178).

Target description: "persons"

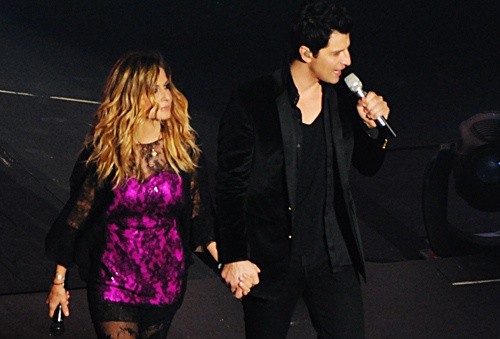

(217, 1), (391, 339)
(46, 50), (255, 339)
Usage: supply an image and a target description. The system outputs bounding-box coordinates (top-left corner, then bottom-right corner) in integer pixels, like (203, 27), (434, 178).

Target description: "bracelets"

(51, 275), (65, 285)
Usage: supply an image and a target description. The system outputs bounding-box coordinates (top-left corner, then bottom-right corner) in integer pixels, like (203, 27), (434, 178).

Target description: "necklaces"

(132, 132), (162, 157)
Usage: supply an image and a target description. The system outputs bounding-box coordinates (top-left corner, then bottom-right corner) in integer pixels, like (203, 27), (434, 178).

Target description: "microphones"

(344, 73), (406, 149)
(50, 304), (65, 336)
